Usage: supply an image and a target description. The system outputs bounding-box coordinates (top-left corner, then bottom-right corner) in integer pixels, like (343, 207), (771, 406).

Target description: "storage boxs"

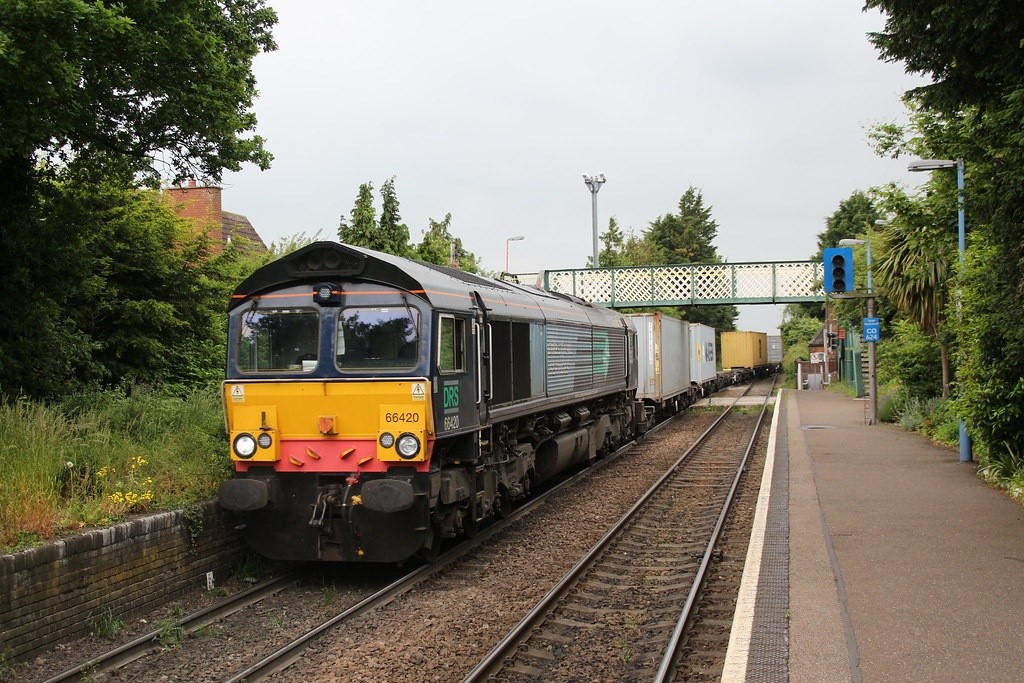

(720, 332), (768, 369)
(627, 311), (691, 404)
(690, 323), (717, 384)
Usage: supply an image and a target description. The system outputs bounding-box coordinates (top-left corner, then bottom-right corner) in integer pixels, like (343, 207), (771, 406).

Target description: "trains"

(219, 239), (784, 580)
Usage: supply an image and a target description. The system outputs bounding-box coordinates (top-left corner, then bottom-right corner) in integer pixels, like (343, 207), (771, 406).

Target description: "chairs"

(398, 340), (417, 360)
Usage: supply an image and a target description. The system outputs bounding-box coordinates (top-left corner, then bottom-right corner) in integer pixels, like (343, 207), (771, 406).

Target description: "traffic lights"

(831, 255), (847, 293)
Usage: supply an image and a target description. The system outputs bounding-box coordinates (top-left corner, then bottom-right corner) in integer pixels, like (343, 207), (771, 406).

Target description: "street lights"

(583, 172), (606, 268)
(838, 239), (881, 426)
(506, 236), (525, 273)
(907, 156), (973, 463)
